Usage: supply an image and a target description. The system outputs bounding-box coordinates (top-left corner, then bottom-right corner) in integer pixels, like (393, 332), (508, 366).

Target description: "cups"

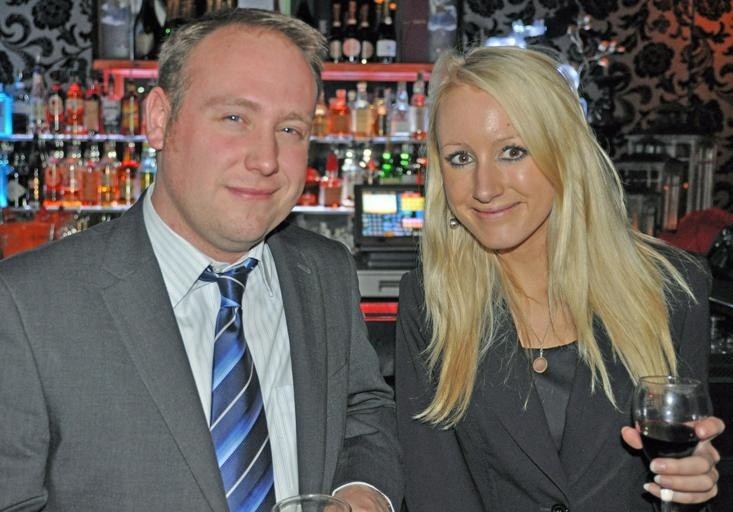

(270, 493), (352, 512)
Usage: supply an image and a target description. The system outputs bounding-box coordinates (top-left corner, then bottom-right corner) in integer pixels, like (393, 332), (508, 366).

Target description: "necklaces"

(530, 323), (552, 373)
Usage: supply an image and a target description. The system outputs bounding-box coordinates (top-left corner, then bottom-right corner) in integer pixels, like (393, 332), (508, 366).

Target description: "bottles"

(0, 0), (431, 211)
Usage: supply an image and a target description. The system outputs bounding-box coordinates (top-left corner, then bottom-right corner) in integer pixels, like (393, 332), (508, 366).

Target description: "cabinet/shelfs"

(0, 59), (435, 214)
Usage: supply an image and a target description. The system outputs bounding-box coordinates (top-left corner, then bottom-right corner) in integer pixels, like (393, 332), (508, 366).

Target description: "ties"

(199, 258), (275, 511)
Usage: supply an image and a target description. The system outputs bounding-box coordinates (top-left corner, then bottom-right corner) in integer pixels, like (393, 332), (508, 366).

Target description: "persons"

(395, 46), (726, 511)
(0, 9), (405, 512)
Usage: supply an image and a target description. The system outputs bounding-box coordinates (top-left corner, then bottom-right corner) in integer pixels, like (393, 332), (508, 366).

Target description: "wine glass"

(631, 373), (707, 512)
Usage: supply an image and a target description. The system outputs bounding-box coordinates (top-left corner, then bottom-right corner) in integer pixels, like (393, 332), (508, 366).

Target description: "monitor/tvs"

(354, 184), (427, 246)
